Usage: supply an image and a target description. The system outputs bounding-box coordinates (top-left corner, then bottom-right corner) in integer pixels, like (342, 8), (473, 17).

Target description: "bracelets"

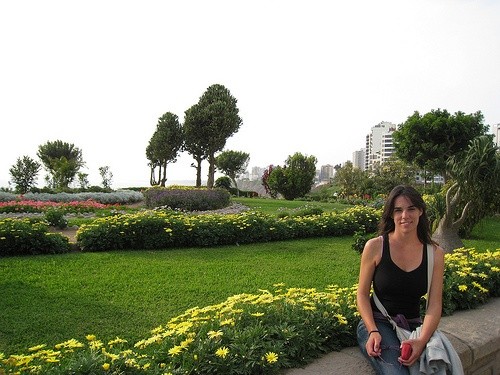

(370, 330), (380, 336)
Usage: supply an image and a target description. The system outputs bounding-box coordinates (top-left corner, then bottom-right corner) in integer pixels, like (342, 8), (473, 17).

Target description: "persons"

(356, 186), (448, 375)
(334, 191), (340, 201)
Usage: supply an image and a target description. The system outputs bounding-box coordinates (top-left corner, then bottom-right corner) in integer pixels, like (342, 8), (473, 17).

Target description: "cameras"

(402, 343), (412, 360)
